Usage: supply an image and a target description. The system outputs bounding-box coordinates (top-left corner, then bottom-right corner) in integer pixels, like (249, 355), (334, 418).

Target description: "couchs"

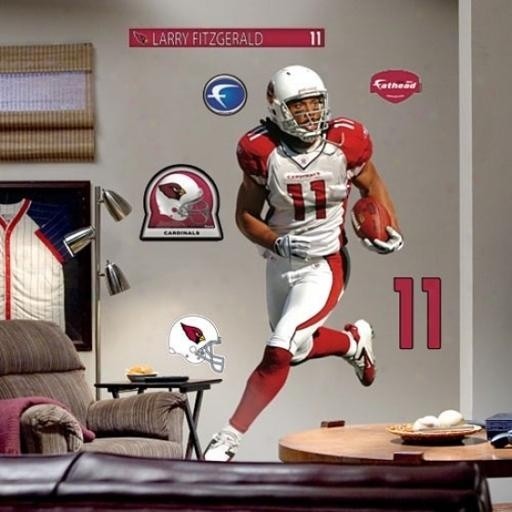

(0, 451), (492, 511)
(0, 318), (187, 459)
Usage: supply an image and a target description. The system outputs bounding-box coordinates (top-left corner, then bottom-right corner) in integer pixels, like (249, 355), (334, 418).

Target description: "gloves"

(357, 225), (405, 255)
(273, 234), (312, 263)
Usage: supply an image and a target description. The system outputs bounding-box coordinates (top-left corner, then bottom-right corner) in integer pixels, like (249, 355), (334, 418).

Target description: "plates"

(127, 374), (157, 382)
(387, 424), (482, 441)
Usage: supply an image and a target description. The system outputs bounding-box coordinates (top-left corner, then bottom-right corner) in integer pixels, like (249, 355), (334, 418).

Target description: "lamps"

(64, 185), (133, 401)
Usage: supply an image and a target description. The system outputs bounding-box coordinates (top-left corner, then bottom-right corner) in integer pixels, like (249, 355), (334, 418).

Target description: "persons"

(200, 64), (404, 463)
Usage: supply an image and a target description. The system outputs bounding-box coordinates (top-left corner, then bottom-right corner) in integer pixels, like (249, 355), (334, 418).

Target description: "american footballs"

(352, 197), (391, 242)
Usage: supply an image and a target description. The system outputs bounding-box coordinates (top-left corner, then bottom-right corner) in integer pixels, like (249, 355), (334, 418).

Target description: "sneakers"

(202, 431), (240, 462)
(343, 319), (376, 387)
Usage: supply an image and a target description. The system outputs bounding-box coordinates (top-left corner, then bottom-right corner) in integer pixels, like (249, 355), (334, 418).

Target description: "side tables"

(94, 373), (222, 461)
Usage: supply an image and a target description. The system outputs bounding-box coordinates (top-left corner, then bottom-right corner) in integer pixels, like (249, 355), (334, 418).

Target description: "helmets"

(168, 314), (224, 373)
(155, 173), (209, 226)
(267, 65), (332, 145)
